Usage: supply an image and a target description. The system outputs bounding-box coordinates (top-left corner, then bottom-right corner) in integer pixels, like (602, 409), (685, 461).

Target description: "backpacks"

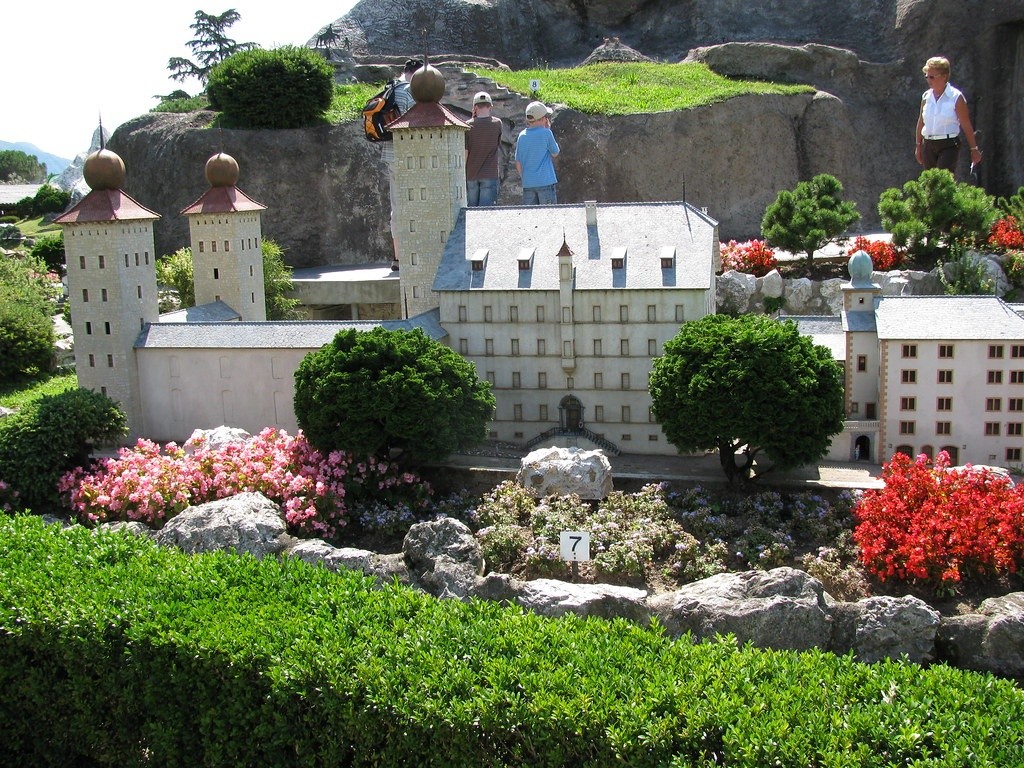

(361, 80), (410, 142)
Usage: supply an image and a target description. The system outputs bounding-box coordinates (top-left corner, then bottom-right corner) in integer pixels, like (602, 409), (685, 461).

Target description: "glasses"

(924, 74), (946, 79)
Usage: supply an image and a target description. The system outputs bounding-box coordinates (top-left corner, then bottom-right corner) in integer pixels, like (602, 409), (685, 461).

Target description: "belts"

(922, 134), (956, 141)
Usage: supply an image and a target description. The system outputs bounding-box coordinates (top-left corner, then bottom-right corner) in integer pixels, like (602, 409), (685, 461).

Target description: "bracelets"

(916, 143), (922, 144)
(969, 145), (978, 151)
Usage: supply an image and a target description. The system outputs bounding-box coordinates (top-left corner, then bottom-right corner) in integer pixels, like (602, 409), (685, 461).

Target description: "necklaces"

(935, 92), (943, 97)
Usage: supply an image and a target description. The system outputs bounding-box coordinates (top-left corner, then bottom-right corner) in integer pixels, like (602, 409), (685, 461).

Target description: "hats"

(526, 101), (553, 122)
(473, 92), (493, 106)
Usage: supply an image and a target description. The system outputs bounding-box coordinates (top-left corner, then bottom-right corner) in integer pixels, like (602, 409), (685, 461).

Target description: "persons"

(342, 38), (352, 50)
(381, 58), (424, 271)
(915, 56), (981, 182)
(515, 101), (560, 205)
(326, 24), (334, 42)
(466, 91), (502, 206)
(855, 445), (860, 460)
